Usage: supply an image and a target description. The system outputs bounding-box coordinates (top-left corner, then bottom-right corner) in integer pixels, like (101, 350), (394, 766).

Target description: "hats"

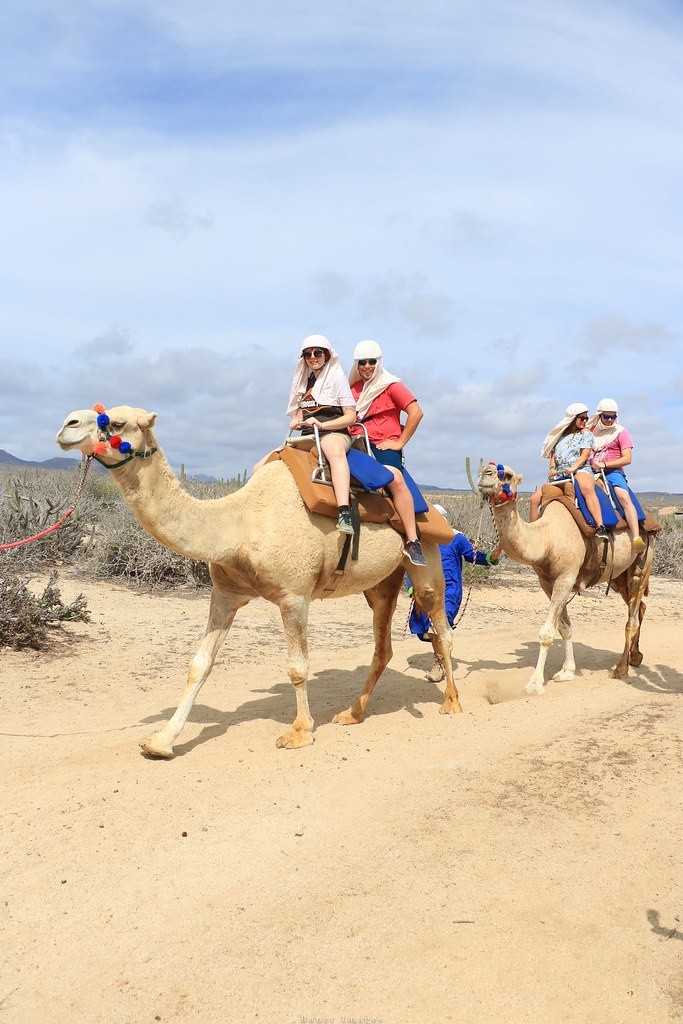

(586, 398), (624, 450)
(540, 403), (589, 458)
(348, 340), (400, 420)
(432, 505), (461, 537)
(286, 335), (356, 415)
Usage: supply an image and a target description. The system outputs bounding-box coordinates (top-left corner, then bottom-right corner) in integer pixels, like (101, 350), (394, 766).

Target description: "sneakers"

(595, 525), (609, 540)
(632, 537), (646, 554)
(336, 513), (355, 535)
(403, 539), (426, 566)
(428, 653), (445, 681)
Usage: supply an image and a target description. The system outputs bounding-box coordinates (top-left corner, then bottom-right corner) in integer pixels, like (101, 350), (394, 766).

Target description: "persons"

(406, 505), (502, 682)
(286, 334), (357, 534)
(529, 403), (609, 538)
(585, 398), (646, 552)
(347, 340), (427, 566)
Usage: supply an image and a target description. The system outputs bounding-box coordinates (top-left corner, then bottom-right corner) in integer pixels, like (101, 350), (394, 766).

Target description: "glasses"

(603, 414), (617, 419)
(304, 351), (323, 359)
(578, 416), (589, 421)
(359, 359), (377, 365)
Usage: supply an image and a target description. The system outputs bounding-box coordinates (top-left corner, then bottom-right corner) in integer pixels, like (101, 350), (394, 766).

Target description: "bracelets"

(318, 422), (323, 430)
(550, 466), (556, 470)
(602, 461), (607, 469)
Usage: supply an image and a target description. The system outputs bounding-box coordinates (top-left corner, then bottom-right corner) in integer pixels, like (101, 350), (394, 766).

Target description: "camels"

(55, 406), (464, 760)
(476, 463), (661, 697)
